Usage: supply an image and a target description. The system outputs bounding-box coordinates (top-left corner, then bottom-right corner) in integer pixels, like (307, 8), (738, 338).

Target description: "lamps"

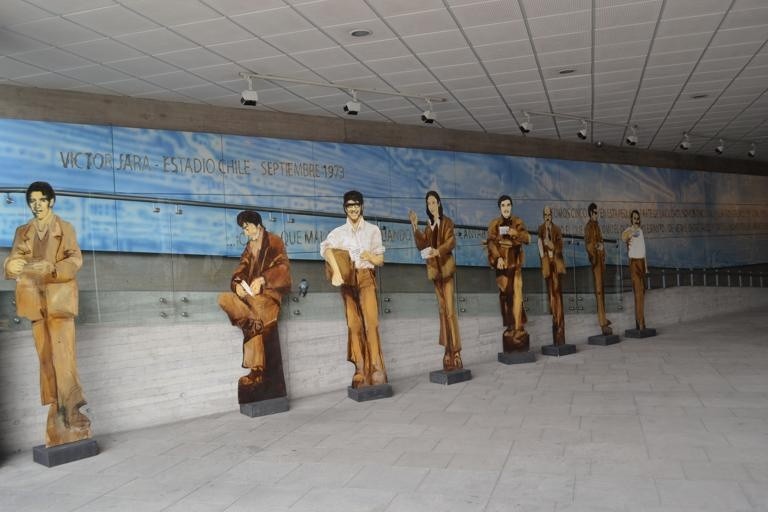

(238, 73), (446, 124)
(518, 110), (638, 146)
(679, 133), (756, 158)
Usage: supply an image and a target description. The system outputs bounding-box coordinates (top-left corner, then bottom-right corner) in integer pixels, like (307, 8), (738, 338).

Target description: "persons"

(315, 189), (389, 390)
(621, 209), (649, 329)
(583, 203), (613, 336)
(218, 209), (291, 406)
(485, 195), (532, 349)
(537, 206), (567, 346)
(2, 181), (90, 446)
(406, 191), (465, 371)
